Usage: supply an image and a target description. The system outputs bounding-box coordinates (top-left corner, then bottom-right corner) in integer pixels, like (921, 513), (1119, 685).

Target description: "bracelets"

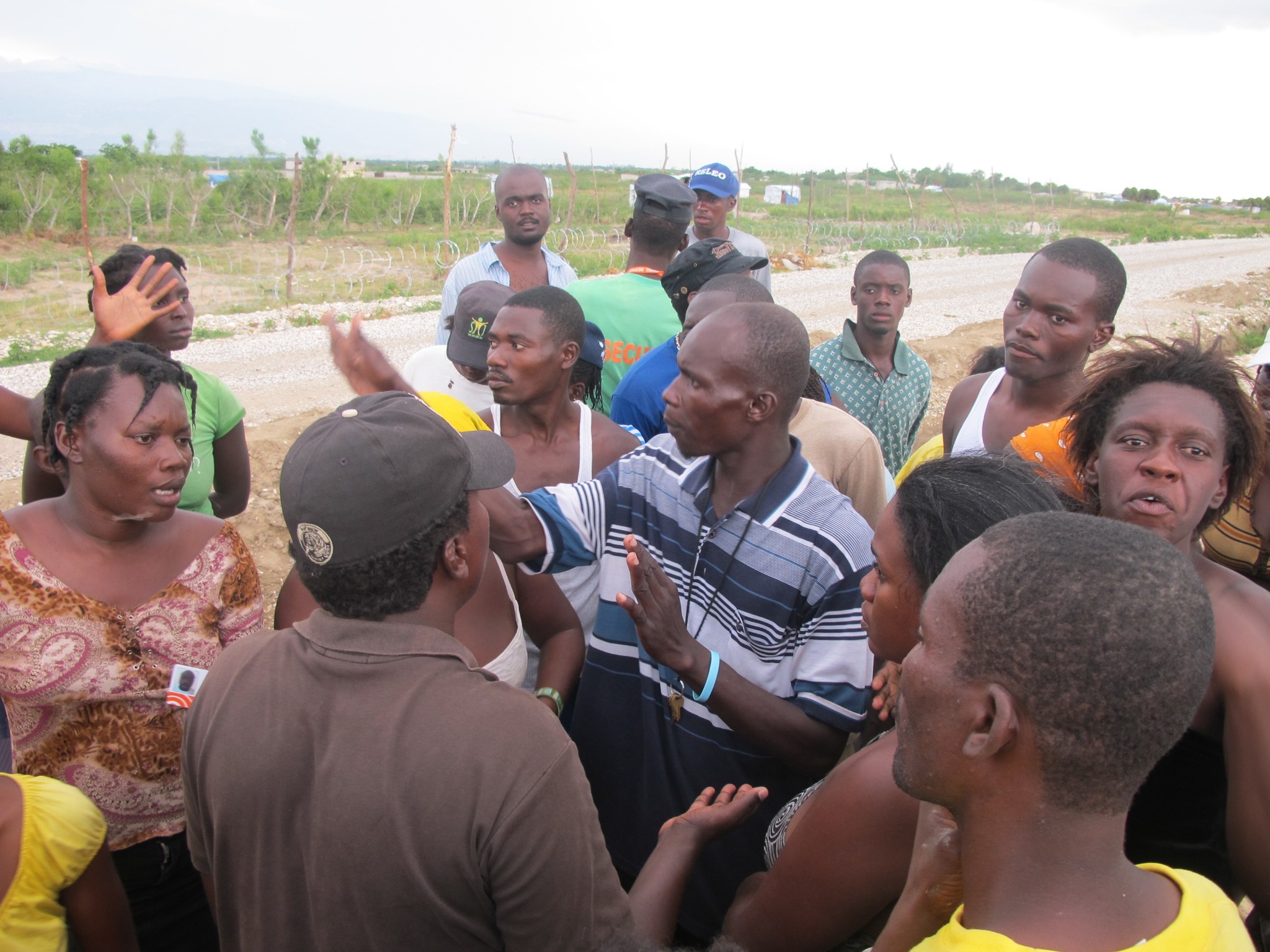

(692, 650), (720, 702)
(533, 687), (564, 719)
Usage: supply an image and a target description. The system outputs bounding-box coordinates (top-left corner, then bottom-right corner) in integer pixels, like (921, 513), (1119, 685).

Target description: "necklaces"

(864, 355), (893, 381)
(676, 331), (682, 351)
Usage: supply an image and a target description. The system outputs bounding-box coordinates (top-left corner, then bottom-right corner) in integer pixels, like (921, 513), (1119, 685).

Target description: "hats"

(447, 276), (515, 366)
(279, 387), (516, 571)
(634, 173), (698, 227)
(576, 319), (604, 367)
(691, 163), (739, 201)
(659, 238), (769, 306)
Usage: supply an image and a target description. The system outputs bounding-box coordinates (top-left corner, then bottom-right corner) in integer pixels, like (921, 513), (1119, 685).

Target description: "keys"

(667, 692), (684, 725)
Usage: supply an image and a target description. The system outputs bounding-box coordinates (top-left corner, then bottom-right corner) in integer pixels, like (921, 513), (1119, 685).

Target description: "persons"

(173, 670), (195, 695)
(0, 771), (140, 952)
(179, 163), (642, 724)
(326, 299), (878, 952)
(0, 340), (266, 952)
(564, 163), (1128, 532)
(711, 318), (1270, 952)
(0, 243), (251, 518)
(179, 392), (768, 952)
